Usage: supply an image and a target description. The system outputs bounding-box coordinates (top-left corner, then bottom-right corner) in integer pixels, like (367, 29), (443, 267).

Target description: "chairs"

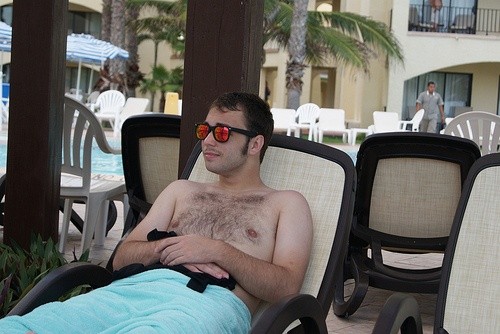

(450, 14), (475, 34)
(408, 7), (433, 31)
(0, 90), (500, 334)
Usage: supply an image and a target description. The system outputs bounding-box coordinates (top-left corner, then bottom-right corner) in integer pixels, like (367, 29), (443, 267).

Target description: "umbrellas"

(66, 32), (130, 95)
(0, 21), (12, 72)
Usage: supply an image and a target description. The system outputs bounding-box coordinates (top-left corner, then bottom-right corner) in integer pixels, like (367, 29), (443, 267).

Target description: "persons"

(0, 92), (312, 334)
(415, 82), (444, 133)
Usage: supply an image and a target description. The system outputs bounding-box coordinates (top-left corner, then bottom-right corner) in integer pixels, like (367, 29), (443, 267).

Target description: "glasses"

(195, 122), (259, 143)
